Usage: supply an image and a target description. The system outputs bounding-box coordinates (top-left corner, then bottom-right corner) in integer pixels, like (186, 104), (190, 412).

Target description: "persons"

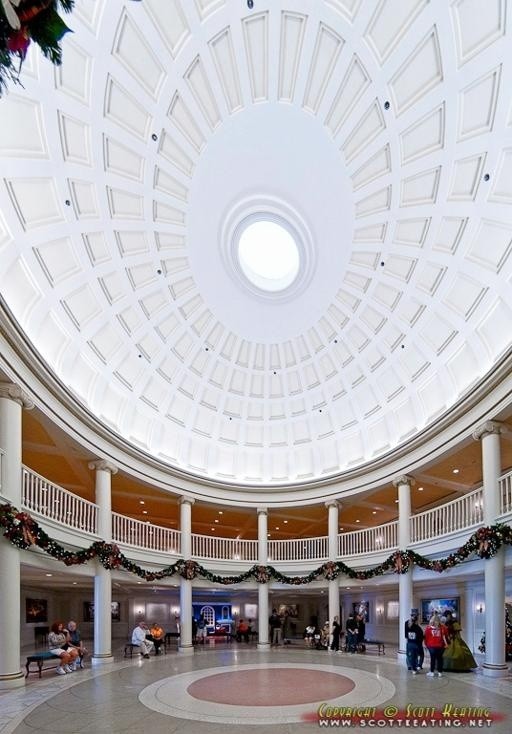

(67, 620), (90, 671)
(175, 608), (478, 677)
(132, 621), (155, 658)
(48, 621), (73, 675)
(148, 623), (166, 657)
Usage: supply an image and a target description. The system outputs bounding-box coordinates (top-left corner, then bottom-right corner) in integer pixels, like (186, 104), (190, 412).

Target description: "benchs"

(360, 639), (386, 656)
(123, 640), (168, 659)
(24, 650), (85, 678)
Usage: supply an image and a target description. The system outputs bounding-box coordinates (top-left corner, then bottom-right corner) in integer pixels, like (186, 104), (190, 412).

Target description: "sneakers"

(412, 667), (442, 677)
(56, 663), (77, 674)
